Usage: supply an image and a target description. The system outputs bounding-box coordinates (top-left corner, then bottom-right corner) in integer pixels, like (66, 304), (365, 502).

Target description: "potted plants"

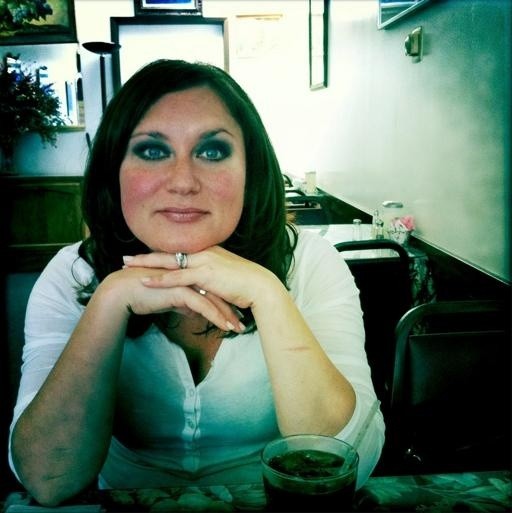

(0, 57), (66, 174)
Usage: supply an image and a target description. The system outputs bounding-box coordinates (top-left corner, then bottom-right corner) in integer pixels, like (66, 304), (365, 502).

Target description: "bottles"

(305, 171), (317, 194)
(352, 200), (404, 241)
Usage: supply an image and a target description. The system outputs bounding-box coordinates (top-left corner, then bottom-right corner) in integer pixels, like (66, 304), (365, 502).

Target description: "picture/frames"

(0, 0), (78, 46)
(307, 2), (329, 90)
(134, 0), (203, 17)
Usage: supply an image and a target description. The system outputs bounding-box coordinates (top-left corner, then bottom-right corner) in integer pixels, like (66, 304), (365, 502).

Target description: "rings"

(199, 287), (207, 295)
(176, 251), (187, 269)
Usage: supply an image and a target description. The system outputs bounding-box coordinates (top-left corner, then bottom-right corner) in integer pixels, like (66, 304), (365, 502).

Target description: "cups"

(263, 434), (359, 513)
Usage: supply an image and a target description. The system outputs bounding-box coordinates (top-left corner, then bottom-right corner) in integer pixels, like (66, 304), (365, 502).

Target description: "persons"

(3, 57), (392, 508)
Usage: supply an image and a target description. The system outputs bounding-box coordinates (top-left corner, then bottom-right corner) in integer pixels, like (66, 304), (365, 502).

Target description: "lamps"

(81, 42), (121, 111)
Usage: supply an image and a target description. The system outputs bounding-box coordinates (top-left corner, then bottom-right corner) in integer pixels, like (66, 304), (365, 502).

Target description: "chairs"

(286, 195), (331, 225)
(336, 239), (414, 311)
(387, 300), (510, 411)
